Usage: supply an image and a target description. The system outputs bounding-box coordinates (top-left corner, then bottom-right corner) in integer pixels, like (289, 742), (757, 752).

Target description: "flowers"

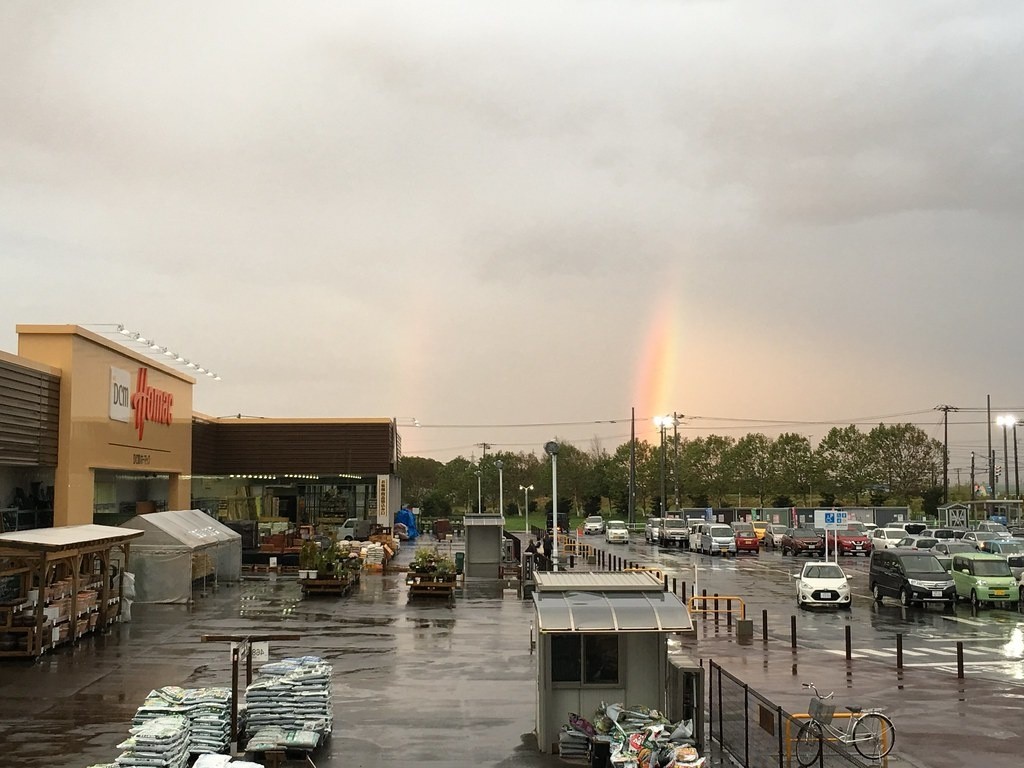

(348, 552), (358, 559)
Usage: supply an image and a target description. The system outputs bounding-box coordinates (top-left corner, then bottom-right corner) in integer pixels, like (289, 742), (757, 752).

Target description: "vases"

(351, 559), (355, 562)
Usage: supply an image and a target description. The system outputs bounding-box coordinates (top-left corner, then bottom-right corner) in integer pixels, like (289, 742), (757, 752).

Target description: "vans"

(701, 524), (737, 557)
(951, 552), (1019, 611)
(869, 549), (957, 608)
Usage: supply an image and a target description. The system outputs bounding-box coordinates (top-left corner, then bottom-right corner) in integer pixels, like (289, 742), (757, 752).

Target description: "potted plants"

(299, 536), (336, 579)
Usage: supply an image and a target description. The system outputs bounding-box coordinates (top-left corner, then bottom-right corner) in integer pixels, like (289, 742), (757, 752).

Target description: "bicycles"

(796, 682), (896, 768)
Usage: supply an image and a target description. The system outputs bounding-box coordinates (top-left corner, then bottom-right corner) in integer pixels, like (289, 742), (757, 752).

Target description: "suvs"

(658, 517), (688, 549)
(546, 513), (570, 534)
(781, 528), (826, 557)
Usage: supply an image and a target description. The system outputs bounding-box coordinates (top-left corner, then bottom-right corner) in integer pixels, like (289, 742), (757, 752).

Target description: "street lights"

(474, 470), (483, 514)
(653, 416), (674, 519)
(996, 414), (1015, 500)
(545, 441), (560, 572)
(519, 484), (534, 534)
(495, 460), (504, 517)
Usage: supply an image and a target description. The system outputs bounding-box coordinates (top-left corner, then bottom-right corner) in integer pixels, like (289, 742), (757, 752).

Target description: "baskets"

(808, 698), (836, 724)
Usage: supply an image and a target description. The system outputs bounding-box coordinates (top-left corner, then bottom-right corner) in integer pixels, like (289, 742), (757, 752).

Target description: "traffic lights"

(994, 465), (1002, 477)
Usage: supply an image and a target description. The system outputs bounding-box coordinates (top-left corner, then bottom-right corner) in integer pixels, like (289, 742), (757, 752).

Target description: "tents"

(117, 508), (243, 605)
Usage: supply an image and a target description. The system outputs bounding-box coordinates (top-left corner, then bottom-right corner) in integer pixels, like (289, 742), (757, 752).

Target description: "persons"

(525, 538), (542, 566)
(542, 533), (554, 561)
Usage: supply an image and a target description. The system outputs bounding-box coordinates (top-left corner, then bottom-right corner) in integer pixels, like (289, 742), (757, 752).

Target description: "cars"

(606, 520), (629, 544)
(793, 560), (853, 612)
(583, 516), (605, 535)
(337, 518), (370, 542)
(644, 517), (1024, 606)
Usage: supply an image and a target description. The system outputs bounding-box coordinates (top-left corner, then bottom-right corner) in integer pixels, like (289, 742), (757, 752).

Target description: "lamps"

(394, 417), (420, 427)
(77, 323), (223, 382)
